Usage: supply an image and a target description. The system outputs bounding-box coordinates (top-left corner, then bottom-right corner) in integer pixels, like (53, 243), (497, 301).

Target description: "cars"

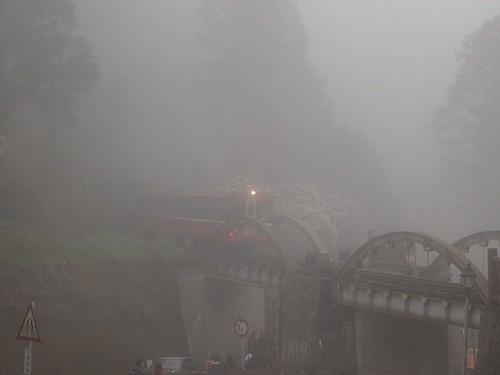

(135, 356), (205, 375)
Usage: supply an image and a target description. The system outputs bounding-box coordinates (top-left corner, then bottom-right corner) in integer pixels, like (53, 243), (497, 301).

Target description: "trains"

(68, 188), (270, 249)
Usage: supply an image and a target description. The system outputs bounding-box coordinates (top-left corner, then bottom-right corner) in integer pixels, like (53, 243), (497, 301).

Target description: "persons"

(130, 328), (271, 375)
